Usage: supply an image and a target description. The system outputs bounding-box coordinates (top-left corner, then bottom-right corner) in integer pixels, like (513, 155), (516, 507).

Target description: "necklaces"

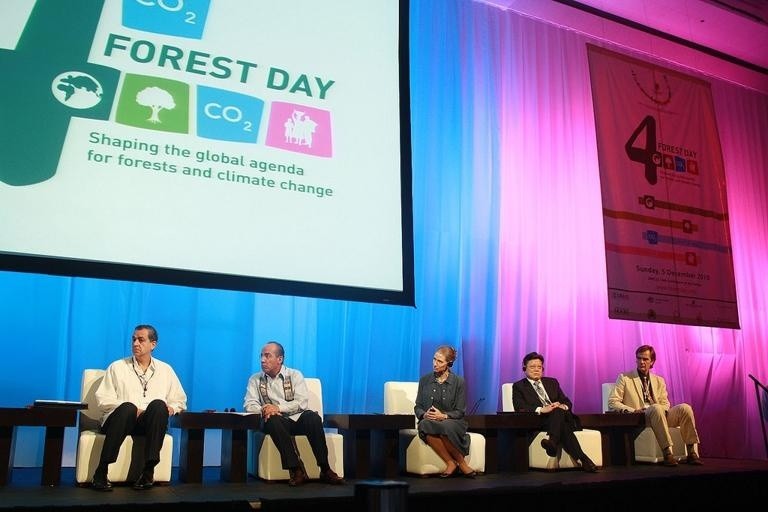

(132, 357), (154, 397)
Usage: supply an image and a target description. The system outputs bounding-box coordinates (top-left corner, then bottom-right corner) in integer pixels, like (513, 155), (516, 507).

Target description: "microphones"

(474, 397), (485, 415)
(469, 398), (482, 415)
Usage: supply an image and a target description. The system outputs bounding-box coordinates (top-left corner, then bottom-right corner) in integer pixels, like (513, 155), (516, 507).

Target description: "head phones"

(447, 345), (455, 367)
(522, 362), (544, 371)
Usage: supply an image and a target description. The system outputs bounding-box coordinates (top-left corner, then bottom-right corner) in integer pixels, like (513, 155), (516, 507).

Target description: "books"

(373, 413), (410, 415)
(34, 400), (81, 405)
(213, 412), (260, 416)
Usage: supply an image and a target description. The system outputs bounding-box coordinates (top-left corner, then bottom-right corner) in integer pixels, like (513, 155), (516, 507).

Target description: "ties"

(534, 381), (551, 405)
(643, 376), (654, 405)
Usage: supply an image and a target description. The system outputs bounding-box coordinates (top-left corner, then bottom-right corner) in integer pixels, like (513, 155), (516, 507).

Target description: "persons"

(512, 352), (597, 473)
(609, 345), (704, 464)
(245, 342), (346, 486)
(91, 325), (187, 491)
(414, 345), (476, 479)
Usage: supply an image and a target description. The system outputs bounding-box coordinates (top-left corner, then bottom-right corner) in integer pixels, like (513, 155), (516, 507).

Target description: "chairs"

(601, 382), (689, 467)
(383, 381), (486, 479)
(247, 378), (345, 485)
(501, 383), (603, 472)
(76, 369), (173, 487)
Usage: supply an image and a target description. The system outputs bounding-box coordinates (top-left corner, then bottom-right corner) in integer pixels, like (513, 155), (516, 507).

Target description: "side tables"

(323, 413), (416, 480)
(576, 413), (644, 470)
(465, 412), (539, 474)
(0, 408), (77, 488)
(170, 412), (260, 483)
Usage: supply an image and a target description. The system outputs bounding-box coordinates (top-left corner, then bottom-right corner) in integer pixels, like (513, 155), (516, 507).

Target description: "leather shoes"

(583, 462), (596, 471)
(289, 471), (307, 486)
(541, 439), (557, 457)
(440, 464), (476, 479)
(91, 478), (112, 491)
(320, 469), (345, 484)
(664, 452), (699, 466)
(134, 474), (153, 489)
